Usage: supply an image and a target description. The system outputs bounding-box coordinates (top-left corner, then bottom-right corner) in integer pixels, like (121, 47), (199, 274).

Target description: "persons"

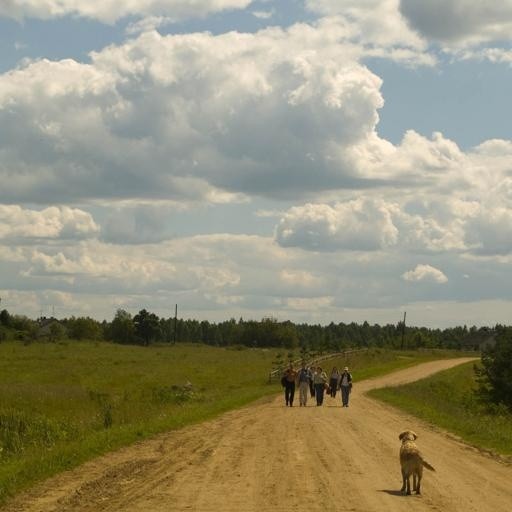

(311, 367), (328, 406)
(296, 361), (313, 407)
(309, 367), (317, 397)
(329, 366), (338, 398)
(338, 366), (353, 407)
(282, 363), (298, 407)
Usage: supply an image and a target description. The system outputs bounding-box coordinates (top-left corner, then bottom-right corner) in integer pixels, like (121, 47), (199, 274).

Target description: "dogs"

(399, 431), (436, 496)
